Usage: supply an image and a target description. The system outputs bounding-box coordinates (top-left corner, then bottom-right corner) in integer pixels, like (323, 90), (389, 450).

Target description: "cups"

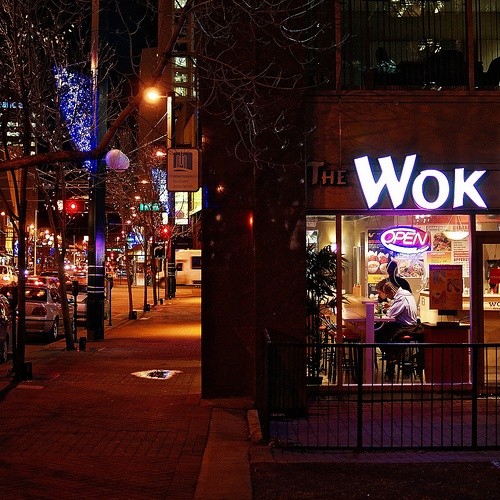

(378, 303), (383, 314)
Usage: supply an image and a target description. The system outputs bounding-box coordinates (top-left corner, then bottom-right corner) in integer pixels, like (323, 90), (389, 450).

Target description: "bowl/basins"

(368, 260), (379, 273)
(380, 263), (388, 274)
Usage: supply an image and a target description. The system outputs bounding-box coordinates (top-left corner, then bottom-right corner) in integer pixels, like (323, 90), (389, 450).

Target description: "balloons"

(106, 149), (130, 172)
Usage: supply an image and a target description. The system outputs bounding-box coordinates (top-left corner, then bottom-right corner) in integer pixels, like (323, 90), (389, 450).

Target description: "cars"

(74, 266), (87, 278)
(117, 266), (127, 277)
(64, 283), (111, 326)
(0, 293), (19, 363)
(38, 271), (73, 288)
(14, 287), (78, 341)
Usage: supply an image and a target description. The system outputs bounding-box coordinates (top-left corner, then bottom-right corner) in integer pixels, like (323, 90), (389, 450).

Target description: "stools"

(317, 321), (423, 384)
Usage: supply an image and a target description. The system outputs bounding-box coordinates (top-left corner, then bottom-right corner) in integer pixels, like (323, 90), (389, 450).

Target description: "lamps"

(443, 216), (469, 240)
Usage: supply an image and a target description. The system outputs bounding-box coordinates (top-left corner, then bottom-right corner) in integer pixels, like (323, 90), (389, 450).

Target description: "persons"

(376, 281), (417, 378)
(375, 261), (413, 359)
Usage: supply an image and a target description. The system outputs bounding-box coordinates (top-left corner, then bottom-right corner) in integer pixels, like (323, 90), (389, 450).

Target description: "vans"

(156, 249), (202, 289)
(0, 265), (19, 287)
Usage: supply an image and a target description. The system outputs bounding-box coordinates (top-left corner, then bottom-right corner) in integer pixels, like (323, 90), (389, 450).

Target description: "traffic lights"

(70, 203), (77, 210)
(163, 227), (168, 233)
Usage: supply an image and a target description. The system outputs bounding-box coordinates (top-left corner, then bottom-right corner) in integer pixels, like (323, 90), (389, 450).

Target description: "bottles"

(353, 283), (361, 298)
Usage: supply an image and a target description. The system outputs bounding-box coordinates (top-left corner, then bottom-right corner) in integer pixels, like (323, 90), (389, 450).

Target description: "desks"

(333, 301), (396, 380)
